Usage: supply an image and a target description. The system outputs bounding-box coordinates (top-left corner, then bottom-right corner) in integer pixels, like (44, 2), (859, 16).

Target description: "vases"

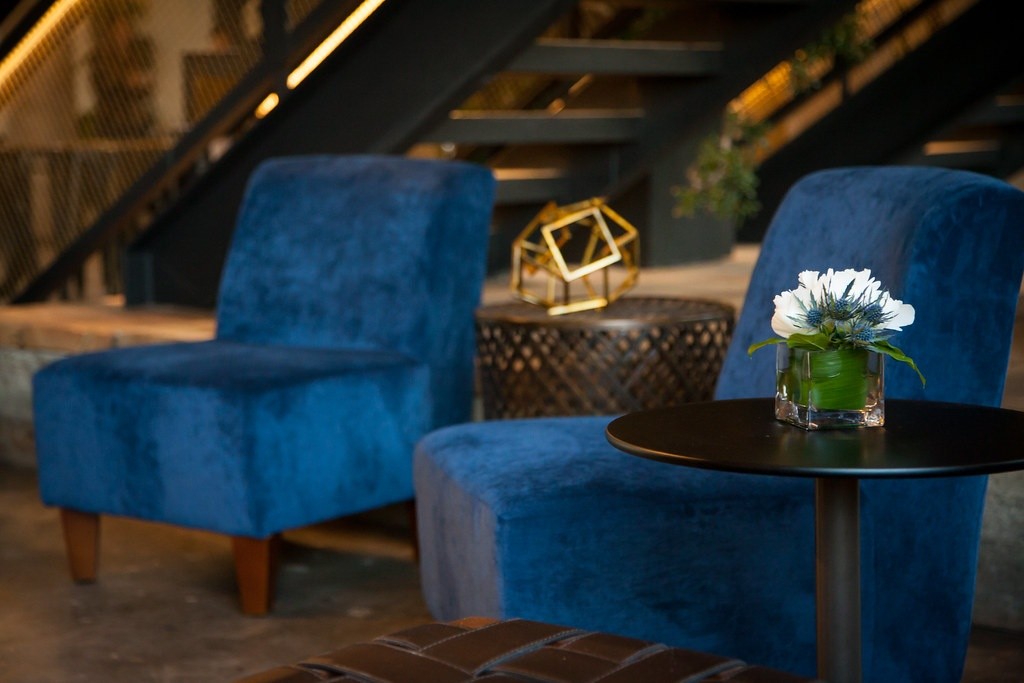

(772, 339), (884, 429)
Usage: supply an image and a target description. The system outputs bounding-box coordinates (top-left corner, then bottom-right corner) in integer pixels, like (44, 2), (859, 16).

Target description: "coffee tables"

(606, 394), (1024, 683)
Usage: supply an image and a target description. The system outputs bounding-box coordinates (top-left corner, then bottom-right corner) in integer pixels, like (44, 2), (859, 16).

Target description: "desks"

(472, 296), (732, 424)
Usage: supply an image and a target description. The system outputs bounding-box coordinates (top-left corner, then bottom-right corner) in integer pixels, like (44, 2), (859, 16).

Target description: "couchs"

(30, 154), (501, 621)
(411, 166), (1024, 680)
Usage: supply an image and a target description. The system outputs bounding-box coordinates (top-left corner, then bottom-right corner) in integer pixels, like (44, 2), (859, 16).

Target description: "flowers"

(746, 265), (927, 410)
